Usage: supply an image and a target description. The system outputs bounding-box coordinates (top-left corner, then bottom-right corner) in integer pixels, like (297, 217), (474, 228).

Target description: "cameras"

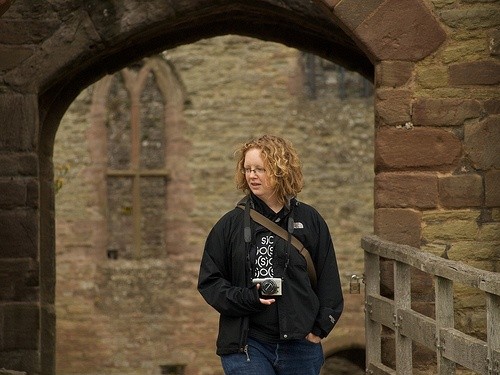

(252, 278), (282, 296)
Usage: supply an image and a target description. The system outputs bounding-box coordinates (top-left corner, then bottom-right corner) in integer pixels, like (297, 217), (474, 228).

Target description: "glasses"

(240, 167), (270, 174)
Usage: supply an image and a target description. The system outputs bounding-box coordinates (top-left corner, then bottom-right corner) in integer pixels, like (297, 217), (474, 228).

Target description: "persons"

(197, 134), (344, 375)
